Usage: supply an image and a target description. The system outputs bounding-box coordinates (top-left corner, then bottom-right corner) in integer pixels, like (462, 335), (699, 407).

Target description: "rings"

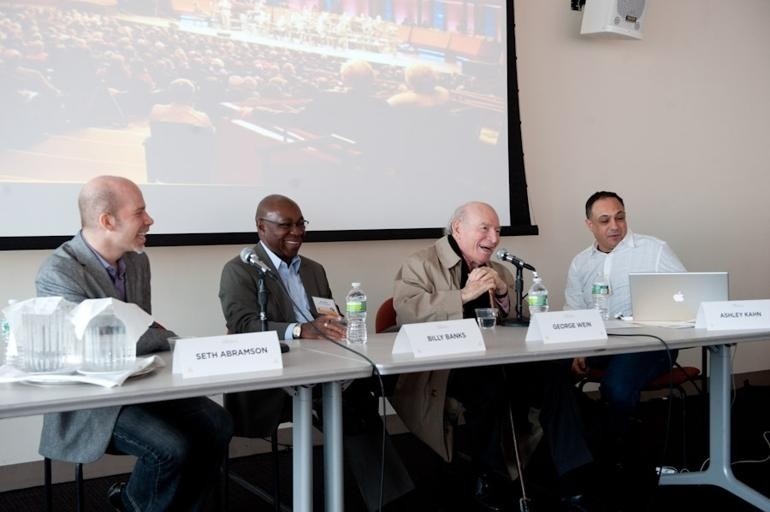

(324, 321), (329, 328)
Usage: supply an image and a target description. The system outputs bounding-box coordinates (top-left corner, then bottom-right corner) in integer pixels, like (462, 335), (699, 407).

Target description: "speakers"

(579, 0), (649, 41)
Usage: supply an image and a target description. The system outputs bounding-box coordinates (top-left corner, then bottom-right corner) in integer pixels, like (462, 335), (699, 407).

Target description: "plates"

(21, 363), (156, 387)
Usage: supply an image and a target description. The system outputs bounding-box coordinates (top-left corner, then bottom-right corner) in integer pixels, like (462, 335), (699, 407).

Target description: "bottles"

(591, 272), (611, 319)
(345, 282), (369, 346)
(527, 273), (549, 315)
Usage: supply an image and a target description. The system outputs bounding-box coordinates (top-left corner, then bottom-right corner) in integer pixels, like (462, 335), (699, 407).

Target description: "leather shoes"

(643, 365), (699, 388)
(475, 476), (506, 510)
(527, 479), (568, 511)
(102, 474), (128, 512)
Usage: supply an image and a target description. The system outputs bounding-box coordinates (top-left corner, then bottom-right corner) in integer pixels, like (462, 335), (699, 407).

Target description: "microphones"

(239, 247), (277, 282)
(496, 248), (536, 273)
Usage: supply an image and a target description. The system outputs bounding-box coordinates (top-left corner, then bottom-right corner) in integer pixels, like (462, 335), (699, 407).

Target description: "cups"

(475, 307), (499, 330)
(166, 337), (197, 358)
(2, 307), (132, 374)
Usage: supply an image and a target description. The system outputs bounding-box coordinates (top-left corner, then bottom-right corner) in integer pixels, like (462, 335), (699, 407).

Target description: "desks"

(280, 319), (769, 512)
(0, 305), (375, 512)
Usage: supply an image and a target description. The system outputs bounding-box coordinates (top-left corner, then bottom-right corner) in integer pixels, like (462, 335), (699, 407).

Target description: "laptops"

(628, 271), (730, 322)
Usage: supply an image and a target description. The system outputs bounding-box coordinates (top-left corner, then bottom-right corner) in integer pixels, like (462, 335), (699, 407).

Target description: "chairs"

(44, 323), (132, 512)
(577, 365), (700, 467)
(376, 297), (396, 332)
(223, 412), (323, 512)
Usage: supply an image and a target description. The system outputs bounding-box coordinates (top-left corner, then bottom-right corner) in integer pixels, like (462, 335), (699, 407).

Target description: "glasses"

(255, 214), (311, 231)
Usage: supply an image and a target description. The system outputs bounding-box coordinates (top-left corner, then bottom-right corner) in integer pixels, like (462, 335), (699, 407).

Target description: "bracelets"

(292, 321), (302, 339)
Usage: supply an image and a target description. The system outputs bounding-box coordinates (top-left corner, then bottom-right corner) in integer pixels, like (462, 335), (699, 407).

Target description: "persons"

(219, 193), (424, 510)
(557, 190), (691, 481)
(31, 173), (234, 509)
(391, 201), (589, 509)
(1, 2), (507, 180)
